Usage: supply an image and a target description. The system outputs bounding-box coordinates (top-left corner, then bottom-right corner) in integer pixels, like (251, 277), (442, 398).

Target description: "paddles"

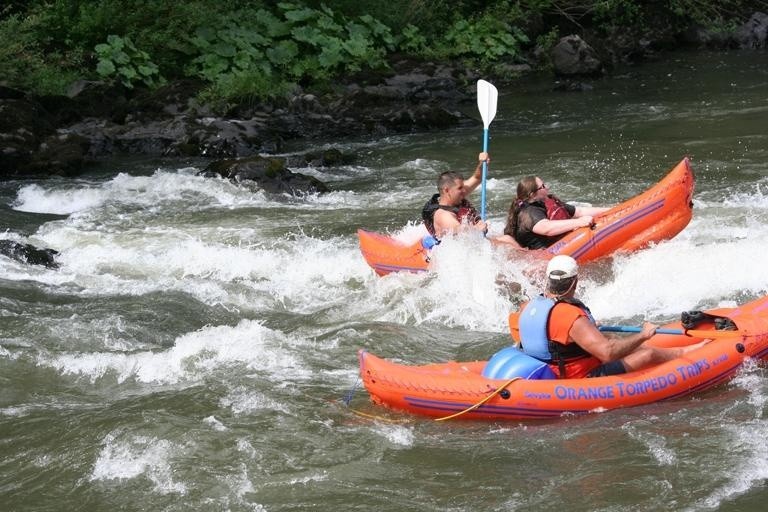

(508, 313), (768, 343)
(477, 79), (498, 223)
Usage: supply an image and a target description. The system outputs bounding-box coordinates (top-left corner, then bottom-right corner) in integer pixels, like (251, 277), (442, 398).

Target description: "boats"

(357, 289), (768, 424)
(352, 155), (698, 286)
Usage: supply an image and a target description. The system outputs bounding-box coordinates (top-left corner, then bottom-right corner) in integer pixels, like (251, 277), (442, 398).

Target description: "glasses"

(535, 184), (545, 192)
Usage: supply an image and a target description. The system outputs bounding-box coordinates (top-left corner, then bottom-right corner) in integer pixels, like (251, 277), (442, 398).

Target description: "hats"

(547, 256), (579, 279)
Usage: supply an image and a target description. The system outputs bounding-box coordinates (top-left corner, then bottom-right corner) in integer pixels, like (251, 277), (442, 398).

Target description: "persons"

(498, 173), (610, 251)
(421, 149), (493, 241)
(515, 254), (717, 380)
